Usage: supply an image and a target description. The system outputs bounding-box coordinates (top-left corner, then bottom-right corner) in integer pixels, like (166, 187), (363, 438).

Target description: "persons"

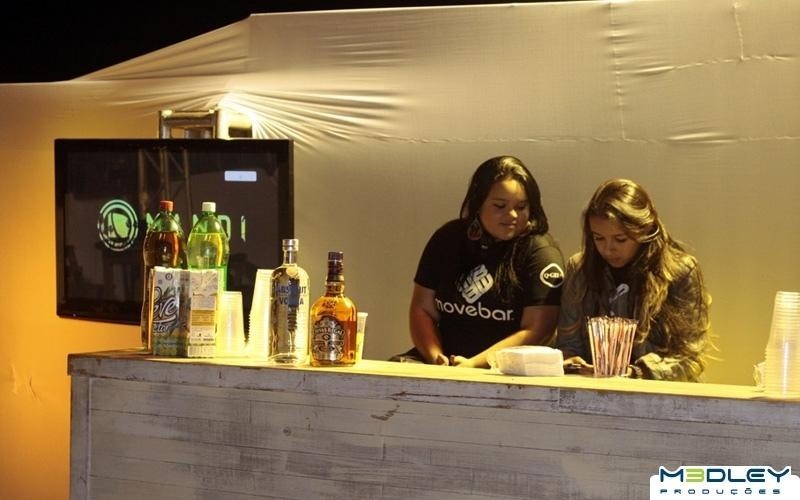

(389, 153), (560, 368)
(553, 177), (710, 385)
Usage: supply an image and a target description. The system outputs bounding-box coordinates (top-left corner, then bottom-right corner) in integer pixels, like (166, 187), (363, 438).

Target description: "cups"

(215, 267), (275, 360)
(356, 311), (369, 363)
(761, 289), (800, 402)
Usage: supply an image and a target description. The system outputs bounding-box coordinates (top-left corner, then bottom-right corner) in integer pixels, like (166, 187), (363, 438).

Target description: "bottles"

(308, 249), (356, 369)
(266, 238), (310, 367)
(138, 199), (230, 351)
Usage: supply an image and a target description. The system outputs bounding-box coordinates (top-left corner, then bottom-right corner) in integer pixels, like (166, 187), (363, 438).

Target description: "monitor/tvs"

(55, 138), (294, 325)
